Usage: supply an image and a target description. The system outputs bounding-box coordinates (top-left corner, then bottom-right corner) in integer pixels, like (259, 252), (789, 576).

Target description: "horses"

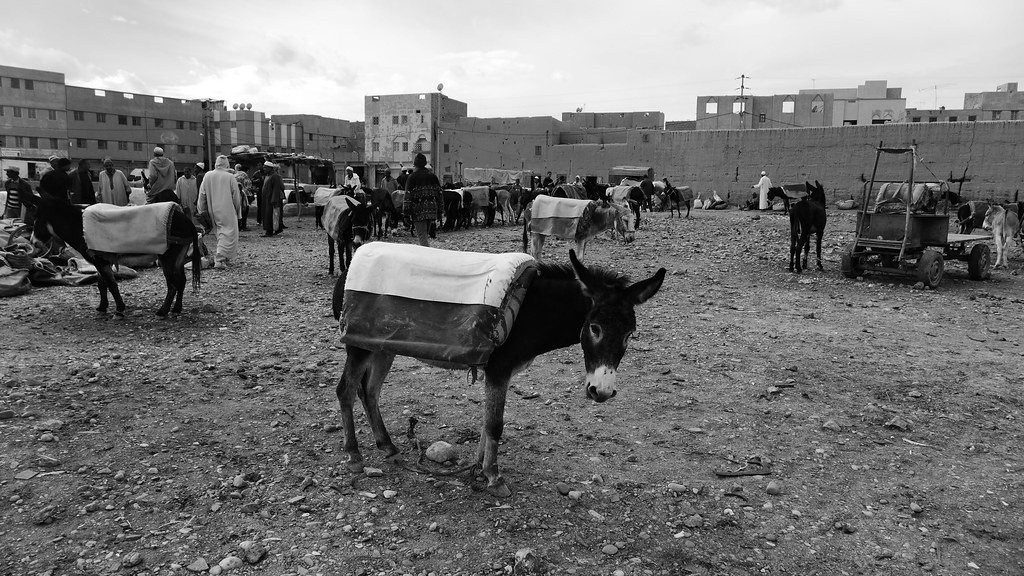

(619, 177), (667, 213)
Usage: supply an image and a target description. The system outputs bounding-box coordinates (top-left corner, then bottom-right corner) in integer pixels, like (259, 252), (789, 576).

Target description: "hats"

(402, 166), (408, 172)
(3, 165), (20, 173)
(104, 158), (113, 164)
(49, 155), (59, 163)
(153, 147), (164, 155)
(264, 160), (275, 166)
(346, 165), (354, 172)
(413, 151), (427, 167)
(197, 161), (205, 169)
(385, 166), (391, 172)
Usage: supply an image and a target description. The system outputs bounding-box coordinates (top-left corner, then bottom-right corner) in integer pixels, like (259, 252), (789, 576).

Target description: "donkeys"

(938, 191), (1024, 270)
(767, 184), (810, 216)
(330, 242), (668, 499)
(521, 194), (636, 262)
(314, 184), (553, 241)
(18, 178), (203, 319)
(666, 185), (693, 219)
(788, 179), (827, 274)
(320, 195), (383, 276)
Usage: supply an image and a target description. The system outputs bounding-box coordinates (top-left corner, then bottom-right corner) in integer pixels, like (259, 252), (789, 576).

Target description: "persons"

(640, 173), (671, 212)
(3, 146), (637, 269)
(403, 153), (445, 247)
(754, 166), (773, 212)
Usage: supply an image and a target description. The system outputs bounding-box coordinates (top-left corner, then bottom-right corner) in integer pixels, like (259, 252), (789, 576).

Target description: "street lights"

(437, 82), (444, 186)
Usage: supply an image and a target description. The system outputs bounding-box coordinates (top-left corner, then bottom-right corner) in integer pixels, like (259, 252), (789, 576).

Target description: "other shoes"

(214, 257), (228, 269)
(259, 232), (271, 238)
(273, 229), (283, 235)
